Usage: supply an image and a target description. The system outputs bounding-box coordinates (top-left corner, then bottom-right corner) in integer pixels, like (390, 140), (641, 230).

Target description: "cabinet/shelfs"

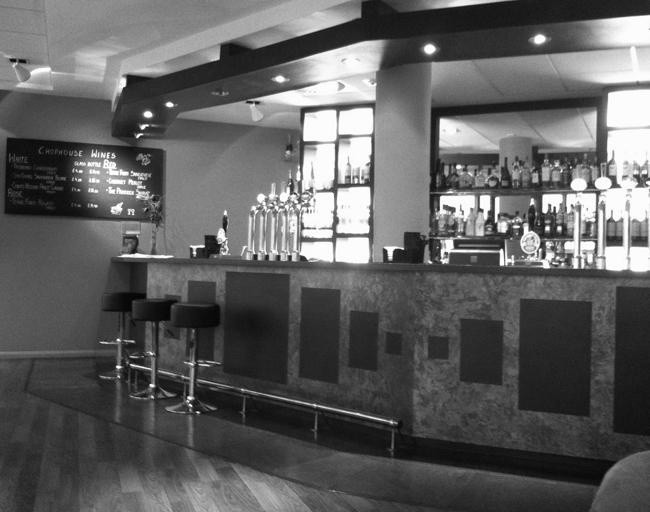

(297, 101), (374, 264)
(428, 174), (650, 248)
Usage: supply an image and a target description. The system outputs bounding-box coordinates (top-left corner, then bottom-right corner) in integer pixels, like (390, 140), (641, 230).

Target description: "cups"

(351, 167), (370, 184)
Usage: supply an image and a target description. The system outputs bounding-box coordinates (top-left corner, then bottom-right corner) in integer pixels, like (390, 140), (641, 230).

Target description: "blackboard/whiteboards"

(4, 138), (164, 222)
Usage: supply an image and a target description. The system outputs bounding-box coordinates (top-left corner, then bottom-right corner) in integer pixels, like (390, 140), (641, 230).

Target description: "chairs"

(587, 450), (650, 512)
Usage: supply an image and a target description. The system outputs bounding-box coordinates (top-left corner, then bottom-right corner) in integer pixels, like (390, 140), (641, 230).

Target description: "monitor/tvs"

(449, 248), (505, 266)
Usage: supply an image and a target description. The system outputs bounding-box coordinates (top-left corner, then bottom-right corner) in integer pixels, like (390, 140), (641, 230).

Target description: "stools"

(98, 292), (146, 381)
(129, 298), (178, 401)
(165, 301), (225, 415)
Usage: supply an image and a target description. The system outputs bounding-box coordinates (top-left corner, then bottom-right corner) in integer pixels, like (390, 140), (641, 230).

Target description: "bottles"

(345, 156), (351, 184)
(431, 150), (650, 191)
(286, 169), (294, 196)
(430, 197), (649, 238)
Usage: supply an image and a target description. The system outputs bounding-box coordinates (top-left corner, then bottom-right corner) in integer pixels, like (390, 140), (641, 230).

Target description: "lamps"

(248, 100), (265, 124)
(10, 59), (31, 83)
